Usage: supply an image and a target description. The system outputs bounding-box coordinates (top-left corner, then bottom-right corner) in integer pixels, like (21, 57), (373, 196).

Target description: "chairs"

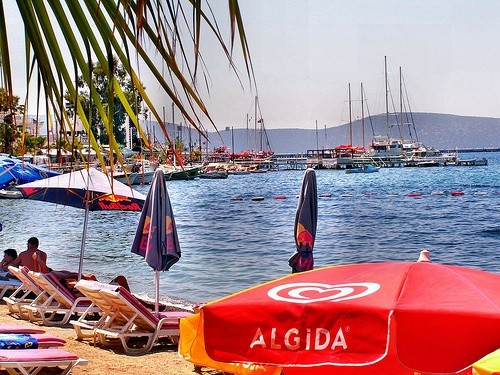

(0, 349), (88, 375)
(0, 266), (194, 356)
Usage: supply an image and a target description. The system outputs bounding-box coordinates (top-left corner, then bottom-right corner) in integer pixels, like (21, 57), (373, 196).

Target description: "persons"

(0, 237), (166, 312)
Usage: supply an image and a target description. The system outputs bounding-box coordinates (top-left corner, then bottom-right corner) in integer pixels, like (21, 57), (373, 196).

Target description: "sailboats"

(0, 55), (459, 199)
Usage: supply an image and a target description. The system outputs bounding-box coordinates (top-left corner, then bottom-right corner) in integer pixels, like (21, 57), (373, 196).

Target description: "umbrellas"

(17, 167), (147, 280)
(131, 170), (181, 313)
(288, 168), (318, 274)
(178, 250), (500, 375)
